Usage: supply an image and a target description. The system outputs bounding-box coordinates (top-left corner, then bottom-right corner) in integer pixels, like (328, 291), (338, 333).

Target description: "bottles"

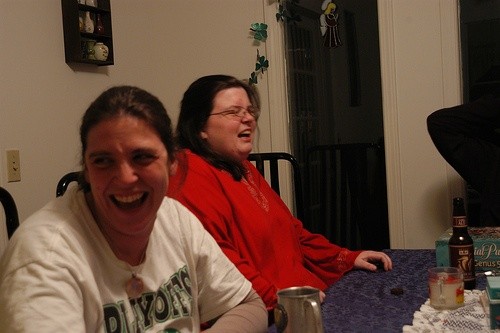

(448, 197), (476, 290)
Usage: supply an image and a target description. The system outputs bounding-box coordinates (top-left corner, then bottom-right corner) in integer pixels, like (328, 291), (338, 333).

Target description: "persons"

(0, 85), (267, 333)
(164, 74), (392, 324)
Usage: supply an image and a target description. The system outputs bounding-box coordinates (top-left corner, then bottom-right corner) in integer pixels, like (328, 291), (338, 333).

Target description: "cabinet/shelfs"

(61, 0), (115, 66)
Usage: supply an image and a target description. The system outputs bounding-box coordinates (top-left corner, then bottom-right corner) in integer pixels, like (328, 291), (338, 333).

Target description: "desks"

(269, 249), (500, 333)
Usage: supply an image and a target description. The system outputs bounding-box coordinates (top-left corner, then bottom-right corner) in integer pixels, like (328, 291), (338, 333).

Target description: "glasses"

(210, 105), (259, 120)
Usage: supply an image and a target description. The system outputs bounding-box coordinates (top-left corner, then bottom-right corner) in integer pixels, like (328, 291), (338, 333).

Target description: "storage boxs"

(435, 227), (500, 275)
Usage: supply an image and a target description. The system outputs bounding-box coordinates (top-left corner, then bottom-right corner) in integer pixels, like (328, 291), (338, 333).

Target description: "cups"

(274, 285), (324, 333)
(429, 266), (465, 310)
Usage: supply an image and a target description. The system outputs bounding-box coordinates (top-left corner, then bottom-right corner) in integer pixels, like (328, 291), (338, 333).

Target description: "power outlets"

(7, 150), (21, 183)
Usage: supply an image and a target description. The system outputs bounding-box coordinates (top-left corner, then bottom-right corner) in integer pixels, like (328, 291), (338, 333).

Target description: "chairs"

(308, 142), (390, 250)
(248, 152), (303, 224)
